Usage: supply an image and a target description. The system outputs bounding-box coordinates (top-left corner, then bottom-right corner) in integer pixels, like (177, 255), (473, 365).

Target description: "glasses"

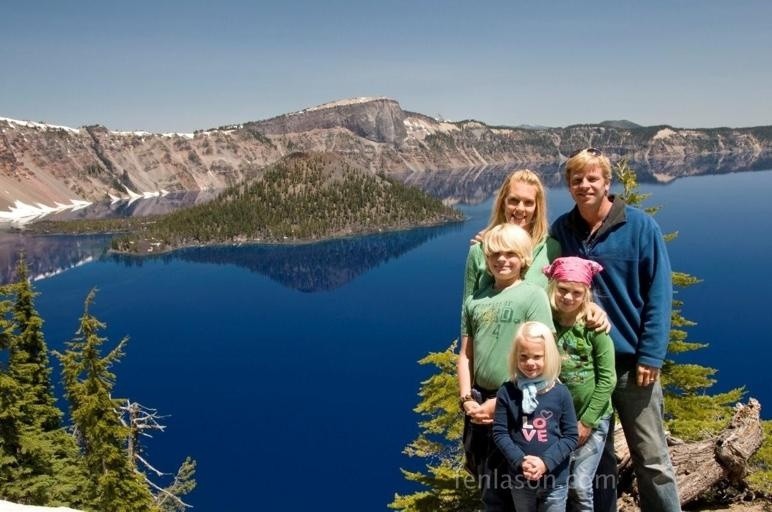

(570, 148), (602, 158)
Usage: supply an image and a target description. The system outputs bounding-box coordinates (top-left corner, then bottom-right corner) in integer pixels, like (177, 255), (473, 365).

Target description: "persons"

(455, 147), (683, 512)
